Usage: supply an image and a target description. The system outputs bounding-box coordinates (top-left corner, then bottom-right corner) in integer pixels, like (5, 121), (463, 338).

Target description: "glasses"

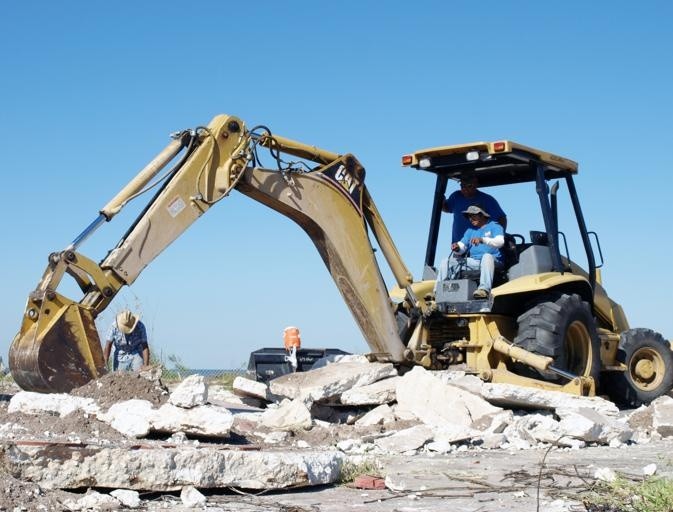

(460, 183), (472, 188)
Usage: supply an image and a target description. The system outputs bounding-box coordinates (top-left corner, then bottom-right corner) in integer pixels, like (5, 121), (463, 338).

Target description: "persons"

(438, 172), (507, 243)
(102, 309), (149, 374)
(423, 200), (505, 301)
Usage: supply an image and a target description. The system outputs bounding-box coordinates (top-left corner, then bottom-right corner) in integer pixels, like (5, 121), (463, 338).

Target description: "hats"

(460, 205), (491, 219)
(111, 308), (141, 335)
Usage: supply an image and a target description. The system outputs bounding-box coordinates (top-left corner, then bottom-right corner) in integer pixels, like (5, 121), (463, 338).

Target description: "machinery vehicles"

(6, 110), (673, 399)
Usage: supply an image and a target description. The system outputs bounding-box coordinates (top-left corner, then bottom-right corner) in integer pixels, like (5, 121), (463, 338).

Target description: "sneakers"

(472, 288), (488, 299)
